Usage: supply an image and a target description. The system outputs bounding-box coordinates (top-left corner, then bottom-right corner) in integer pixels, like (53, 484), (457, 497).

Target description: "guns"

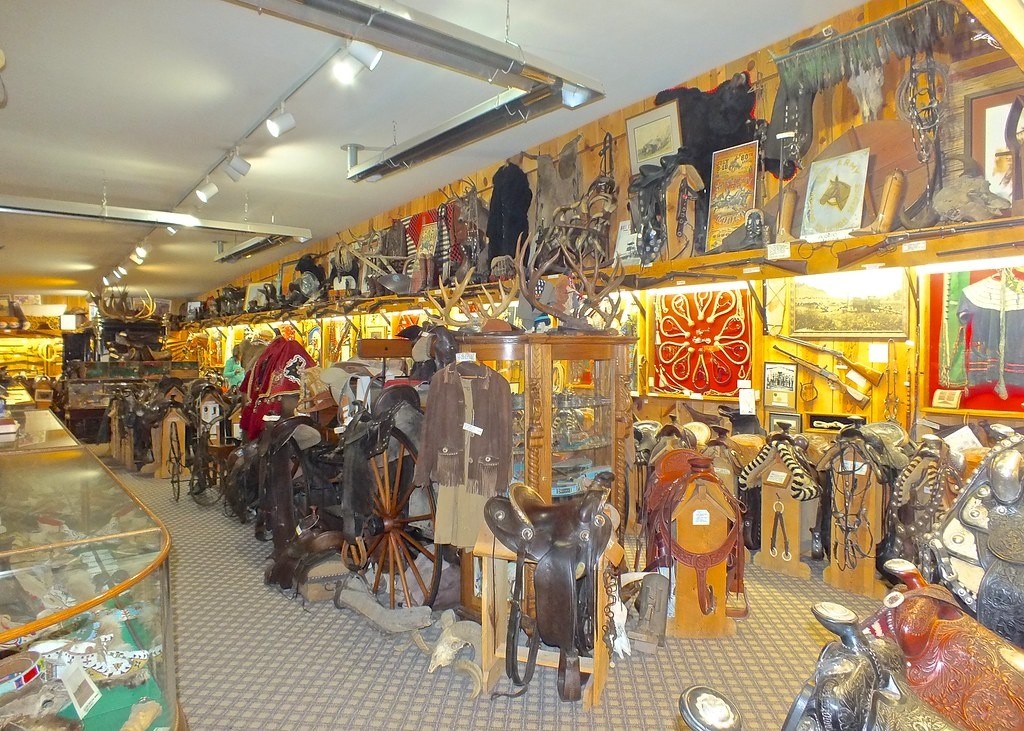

(316, 308), (345, 317)
(397, 292), (445, 308)
(936, 240), (1024, 256)
(344, 299), (374, 313)
(178, 303), (333, 330)
(688, 255), (808, 274)
(776, 335), (883, 387)
(773, 344), (870, 410)
(462, 291), (495, 299)
(837, 218), (1024, 268)
(464, 284), (519, 298)
(366, 299), (415, 313)
(572, 283), (606, 294)
(570, 271), (636, 289)
(638, 271), (738, 288)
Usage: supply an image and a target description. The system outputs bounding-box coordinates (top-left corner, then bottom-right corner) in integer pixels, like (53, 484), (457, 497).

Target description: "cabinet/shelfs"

(460, 333), (640, 633)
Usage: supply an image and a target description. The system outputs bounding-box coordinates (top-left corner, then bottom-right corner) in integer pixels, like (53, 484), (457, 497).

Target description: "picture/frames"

(800, 146), (870, 240)
(645, 281), (756, 403)
(412, 222), (438, 270)
(245, 282), (271, 311)
(587, 291), (642, 398)
(768, 411), (803, 435)
(789, 268), (911, 337)
(763, 361), (798, 411)
(367, 326), (388, 339)
(625, 98), (684, 175)
(963, 81), (1024, 219)
(705, 140), (758, 252)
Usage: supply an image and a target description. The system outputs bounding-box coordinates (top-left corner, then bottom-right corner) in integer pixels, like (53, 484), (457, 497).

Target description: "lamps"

(91, 289), (99, 298)
(136, 239), (153, 257)
(223, 153), (242, 183)
(229, 146), (252, 175)
(165, 227), (181, 236)
(195, 174), (219, 204)
(129, 253), (144, 266)
(102, 262), (132, 287)
(267, 100), (296, 138)
(349, 39), (384, 70)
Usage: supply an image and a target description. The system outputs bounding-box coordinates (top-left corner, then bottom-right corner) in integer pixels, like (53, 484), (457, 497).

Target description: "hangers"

(992, 268), (1001, 278)
(456, 332), (487, 378)
(501, 158), (510, 170)
